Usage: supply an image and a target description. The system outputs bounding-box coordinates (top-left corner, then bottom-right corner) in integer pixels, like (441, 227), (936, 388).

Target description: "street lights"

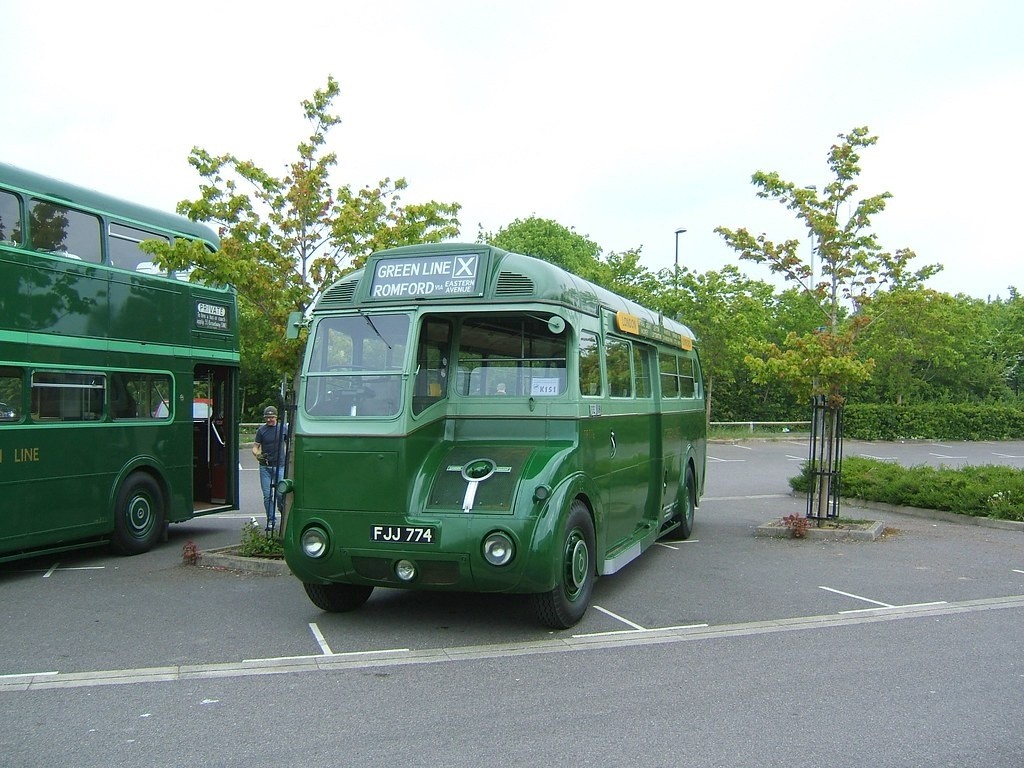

(672, 227), (688, 299)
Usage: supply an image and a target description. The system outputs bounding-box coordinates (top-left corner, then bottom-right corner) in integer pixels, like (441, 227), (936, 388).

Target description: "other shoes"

(265, 520), (276, 530)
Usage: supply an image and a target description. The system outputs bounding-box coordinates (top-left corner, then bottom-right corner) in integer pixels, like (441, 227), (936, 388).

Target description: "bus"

(0, 162), (247, 563)
(277, 241), (710, 628)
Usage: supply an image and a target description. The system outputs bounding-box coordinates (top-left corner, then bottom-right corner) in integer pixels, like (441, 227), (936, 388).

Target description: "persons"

(252, 406), (287, 530)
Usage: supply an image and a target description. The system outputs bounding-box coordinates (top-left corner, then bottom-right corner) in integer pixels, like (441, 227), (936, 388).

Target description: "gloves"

(256, 454), (268, 463)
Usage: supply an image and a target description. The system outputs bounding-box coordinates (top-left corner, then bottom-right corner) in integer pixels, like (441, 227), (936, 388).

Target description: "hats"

(263, 406), (278, 418)
